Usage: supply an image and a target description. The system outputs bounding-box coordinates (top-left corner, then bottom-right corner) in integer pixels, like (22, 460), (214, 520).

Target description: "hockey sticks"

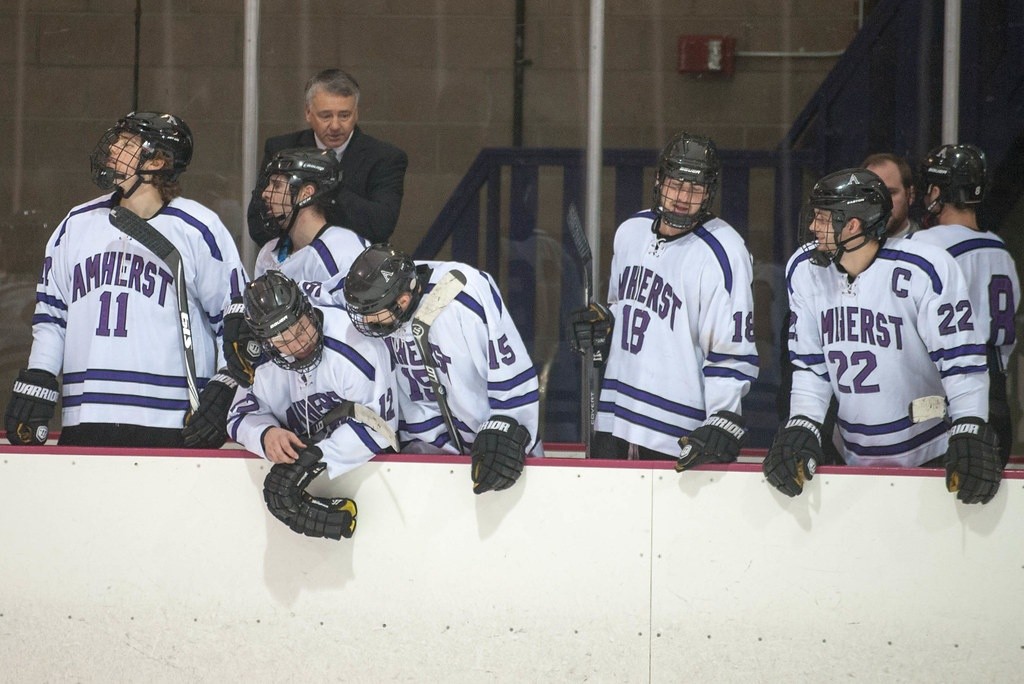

(565, 193), (603, 457)
(300, 400), (401, 454)
(107, 207), (198, 416)
(409, 270), (466, 454)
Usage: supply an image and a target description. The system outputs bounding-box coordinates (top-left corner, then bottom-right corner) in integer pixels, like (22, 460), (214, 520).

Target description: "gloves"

(4, 367), (58, 448)
(221, 307), (261, 390)
(672, 410), (750, 472)
(942, 415), (1008, 506)
(761, 421), (828, 499)
(262, 444), (358, 541)
(564, 304), (614, 362)
(469, 415), (532, 497)
(178, 369), (246, 451)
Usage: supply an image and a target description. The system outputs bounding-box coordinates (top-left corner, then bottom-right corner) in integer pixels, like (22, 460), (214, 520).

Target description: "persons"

(4, 110), (251, 449)
(225, 268), (399, 539)
(762, 166), (1004, 505)
(565, 131), (760, 472)
(253, 147), (372, 283)
(773, 153), (931, 466)
(900, 143), (1021, 470)
(222, 243), (546, 495)
(246, 71), (408, 248)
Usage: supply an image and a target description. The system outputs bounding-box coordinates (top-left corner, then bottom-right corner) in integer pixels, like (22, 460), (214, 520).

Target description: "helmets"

(242, 267), (323, 374)
(253, 146), (344, 232)
(913, 142), (989, 221)
(89, 109), (194, 189)
(799, 165), (893, 268)
(342, 242), (420, 339)
(650, 129), (721, 229)
